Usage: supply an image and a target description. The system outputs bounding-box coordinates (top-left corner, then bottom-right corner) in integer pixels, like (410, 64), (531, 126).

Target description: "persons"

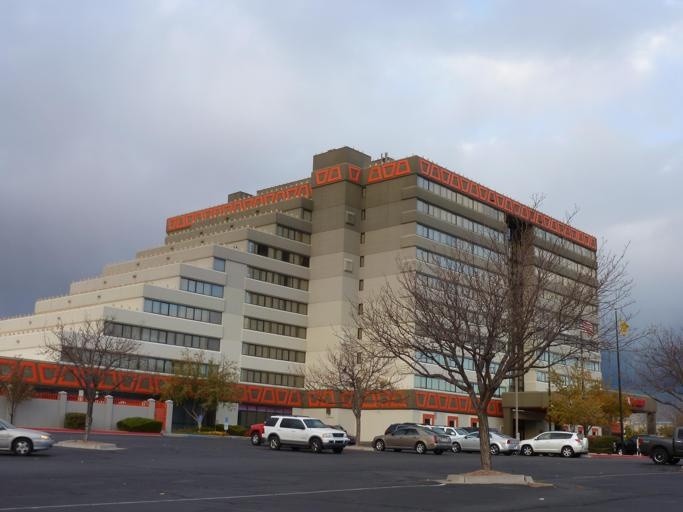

(636, 436), (642, 456)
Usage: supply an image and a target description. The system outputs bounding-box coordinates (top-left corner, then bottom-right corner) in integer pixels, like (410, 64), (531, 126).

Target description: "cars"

(371, 423), (517, 455)
(0, 419), (55, 456)
(612, 434), (657, 456)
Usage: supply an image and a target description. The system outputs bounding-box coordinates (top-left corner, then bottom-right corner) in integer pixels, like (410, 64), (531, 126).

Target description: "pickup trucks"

(636, 425), (683, 465)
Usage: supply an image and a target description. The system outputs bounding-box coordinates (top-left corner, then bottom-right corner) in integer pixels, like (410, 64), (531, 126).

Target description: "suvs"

(518, 431), (589, 458)
(248, 415), (355, 454)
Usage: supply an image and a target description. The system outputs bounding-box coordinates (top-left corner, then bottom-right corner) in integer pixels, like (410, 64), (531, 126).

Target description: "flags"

(618, 319), (630, 336)
